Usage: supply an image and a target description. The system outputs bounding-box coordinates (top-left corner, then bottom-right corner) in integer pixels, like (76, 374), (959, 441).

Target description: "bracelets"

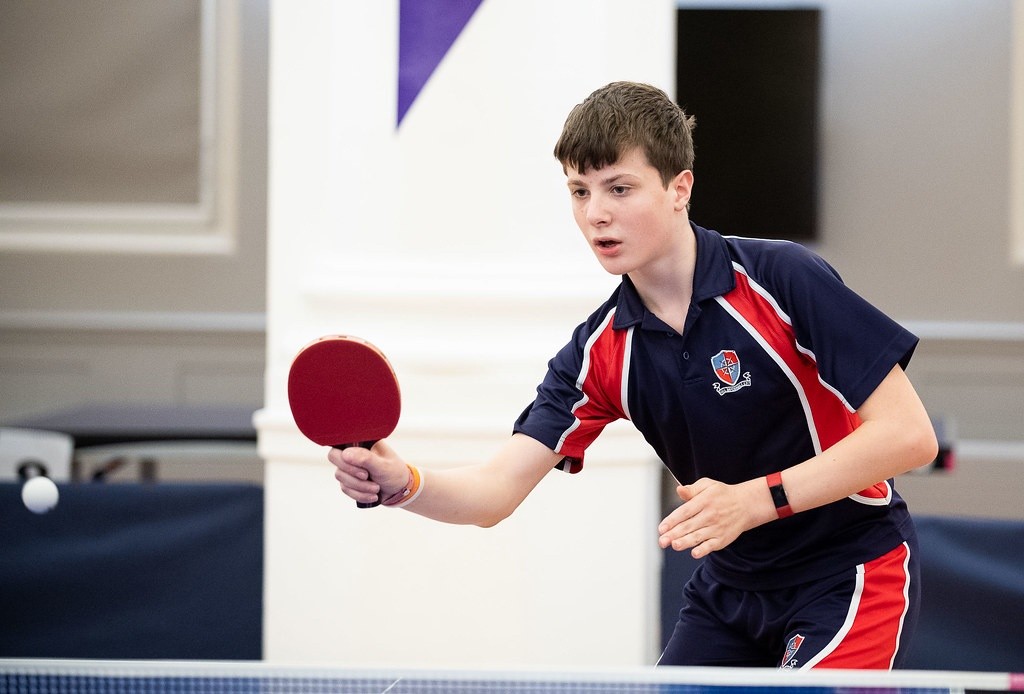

(378, 462), (425, 513)
(764, 472), (797, 523)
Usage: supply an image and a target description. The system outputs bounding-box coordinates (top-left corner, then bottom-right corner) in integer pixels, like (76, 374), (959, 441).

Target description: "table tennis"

(20, 477), (60, 513)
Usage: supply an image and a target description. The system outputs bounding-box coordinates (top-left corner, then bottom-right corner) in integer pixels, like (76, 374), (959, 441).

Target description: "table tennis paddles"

(287, 337), (402, 509)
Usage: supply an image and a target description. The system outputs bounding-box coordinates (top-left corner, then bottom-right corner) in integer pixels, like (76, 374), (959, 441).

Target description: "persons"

(324, 81), (945, 670)
